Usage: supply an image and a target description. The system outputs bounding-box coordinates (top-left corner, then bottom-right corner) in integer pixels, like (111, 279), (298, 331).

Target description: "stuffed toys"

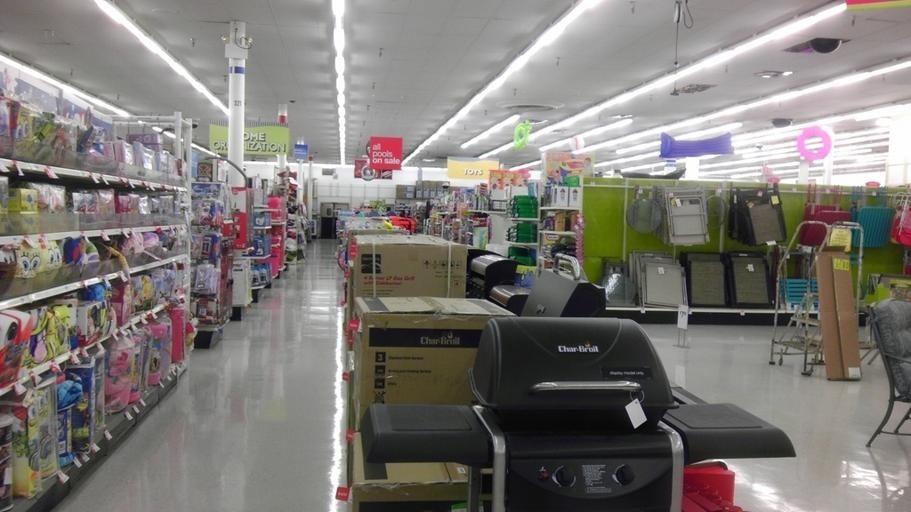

(1, 90), (191, 512)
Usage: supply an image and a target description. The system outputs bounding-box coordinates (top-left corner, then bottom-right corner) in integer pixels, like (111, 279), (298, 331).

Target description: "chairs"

(865, 298), (911, 447)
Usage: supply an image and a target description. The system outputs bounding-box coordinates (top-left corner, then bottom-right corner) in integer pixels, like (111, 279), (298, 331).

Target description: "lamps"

(1, 0), (911, 175)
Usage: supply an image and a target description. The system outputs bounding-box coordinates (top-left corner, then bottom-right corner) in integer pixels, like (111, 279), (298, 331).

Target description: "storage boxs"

(343, 227), (517, 432)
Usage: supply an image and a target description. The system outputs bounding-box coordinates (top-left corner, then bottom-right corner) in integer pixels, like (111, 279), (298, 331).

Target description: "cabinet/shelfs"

(417, 148), (911, 317)
(0, 58), (316, 512)
